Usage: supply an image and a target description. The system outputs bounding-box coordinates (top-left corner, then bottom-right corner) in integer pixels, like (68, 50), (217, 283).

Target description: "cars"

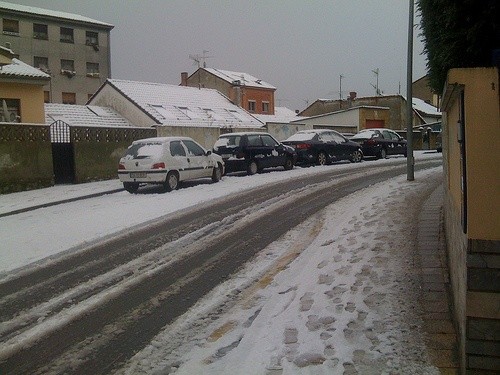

(213, 131), (296, 175)
(279, 129), (364, 168)
(349, 127), (413, 159)
(117, 137), (226, 193)
